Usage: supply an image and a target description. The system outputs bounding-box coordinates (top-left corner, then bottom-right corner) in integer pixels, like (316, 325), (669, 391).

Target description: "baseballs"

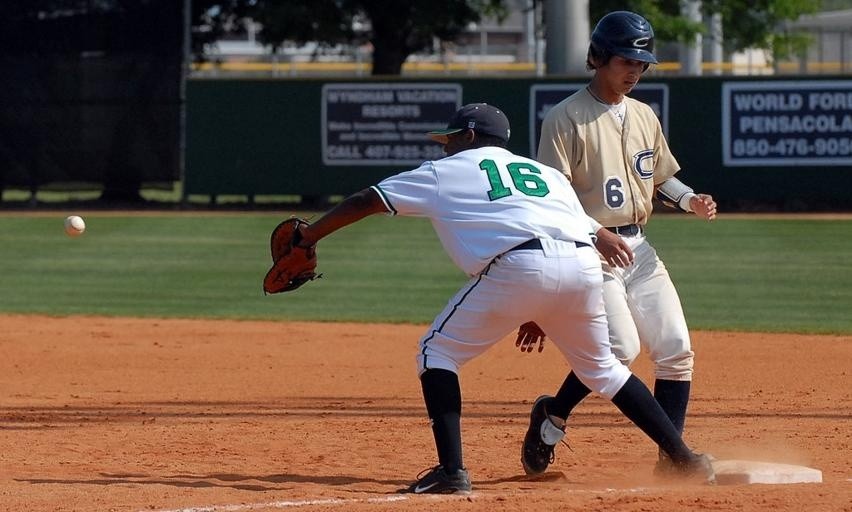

(64, 216), (85, 237)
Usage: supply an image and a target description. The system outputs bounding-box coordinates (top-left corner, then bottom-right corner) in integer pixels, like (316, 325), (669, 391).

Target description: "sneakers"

(521, 395), (567, 477)
(650, 446), (725, 488)
(388, 464), (472, 496)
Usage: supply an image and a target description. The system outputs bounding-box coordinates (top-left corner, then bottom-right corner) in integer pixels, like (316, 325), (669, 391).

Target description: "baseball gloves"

(262, 216), (318, 295)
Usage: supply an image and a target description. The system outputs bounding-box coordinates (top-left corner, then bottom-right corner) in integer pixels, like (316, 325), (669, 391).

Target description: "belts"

(501, 237), (593, 251)
(604, 223), (646, 237)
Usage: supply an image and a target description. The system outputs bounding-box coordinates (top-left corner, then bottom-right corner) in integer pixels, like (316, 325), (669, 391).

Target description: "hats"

(424, 102), (513, 146)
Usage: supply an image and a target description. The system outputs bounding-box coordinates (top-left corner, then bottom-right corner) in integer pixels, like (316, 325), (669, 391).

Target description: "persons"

(520, 10), (719, 476)
(263, 101), (720, 493)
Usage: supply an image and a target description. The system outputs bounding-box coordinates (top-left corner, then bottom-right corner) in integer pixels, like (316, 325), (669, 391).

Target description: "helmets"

(590, 9), (659, 73)
